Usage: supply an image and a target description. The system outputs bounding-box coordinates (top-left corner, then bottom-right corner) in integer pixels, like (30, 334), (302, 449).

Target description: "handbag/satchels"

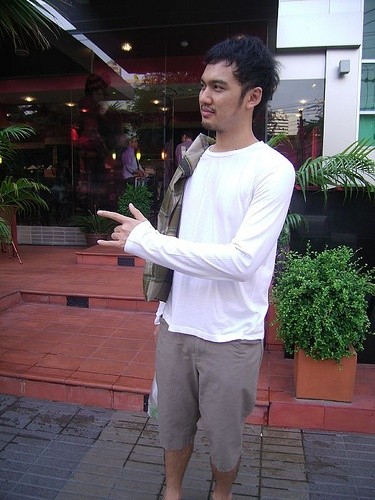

(142, 132), (217, 303)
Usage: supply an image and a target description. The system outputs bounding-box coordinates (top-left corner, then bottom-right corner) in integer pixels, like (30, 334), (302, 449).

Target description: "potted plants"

(76, 204), (119, 247)
(269, 240), (375, 403)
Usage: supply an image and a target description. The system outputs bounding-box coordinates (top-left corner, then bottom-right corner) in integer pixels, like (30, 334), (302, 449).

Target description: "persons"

(175, 128), (194, 166)
(121, 131), (149, 190)
(94, 32), (297, 499)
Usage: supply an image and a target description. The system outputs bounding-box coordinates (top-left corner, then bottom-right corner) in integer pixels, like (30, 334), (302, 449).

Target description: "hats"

(120, 131), (138, 147)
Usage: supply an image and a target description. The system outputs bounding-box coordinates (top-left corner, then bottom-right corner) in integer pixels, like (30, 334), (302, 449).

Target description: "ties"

(134, 152), (140, 169)
